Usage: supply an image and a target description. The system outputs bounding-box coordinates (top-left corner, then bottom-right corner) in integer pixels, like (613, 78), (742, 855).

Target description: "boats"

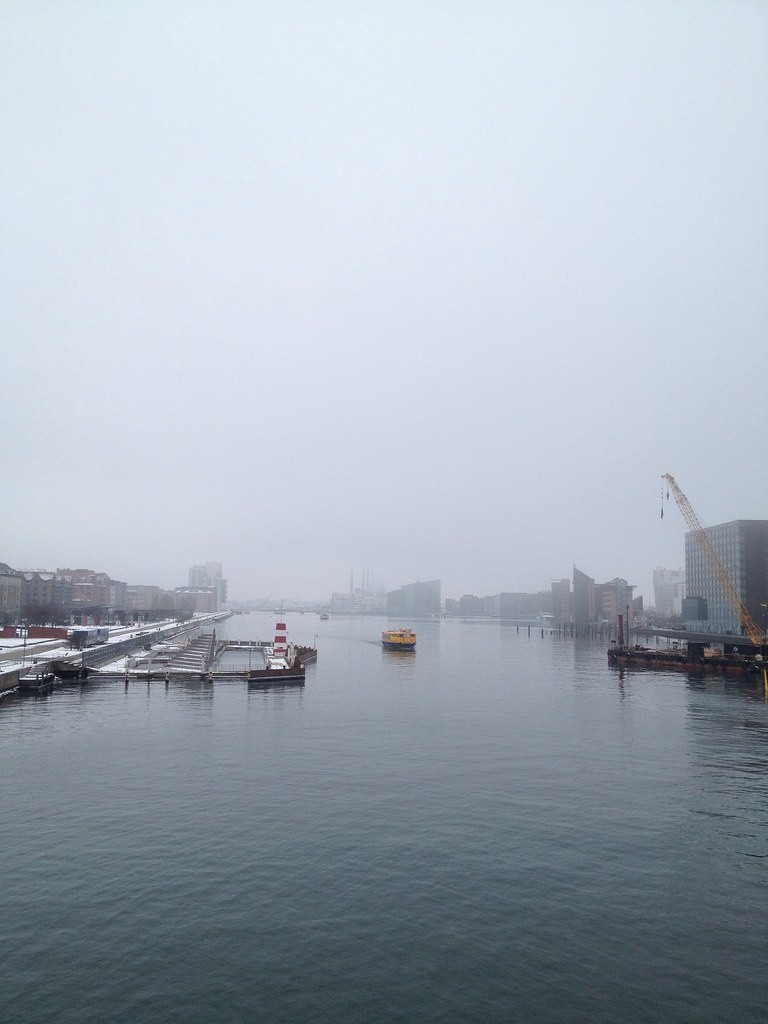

(320, 613), (329, 620)
(381, 627), (416, 651)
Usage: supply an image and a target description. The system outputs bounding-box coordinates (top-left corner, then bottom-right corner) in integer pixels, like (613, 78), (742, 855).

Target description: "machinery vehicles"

(606, 472), (768, 676)
